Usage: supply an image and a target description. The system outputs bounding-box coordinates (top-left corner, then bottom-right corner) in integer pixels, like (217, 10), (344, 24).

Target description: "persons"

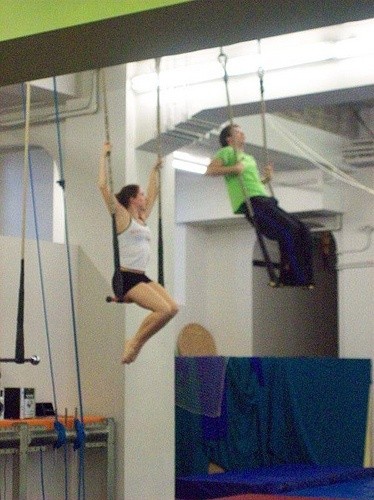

(97, 139), (180, 366)
(206, 123), (314, 287)
